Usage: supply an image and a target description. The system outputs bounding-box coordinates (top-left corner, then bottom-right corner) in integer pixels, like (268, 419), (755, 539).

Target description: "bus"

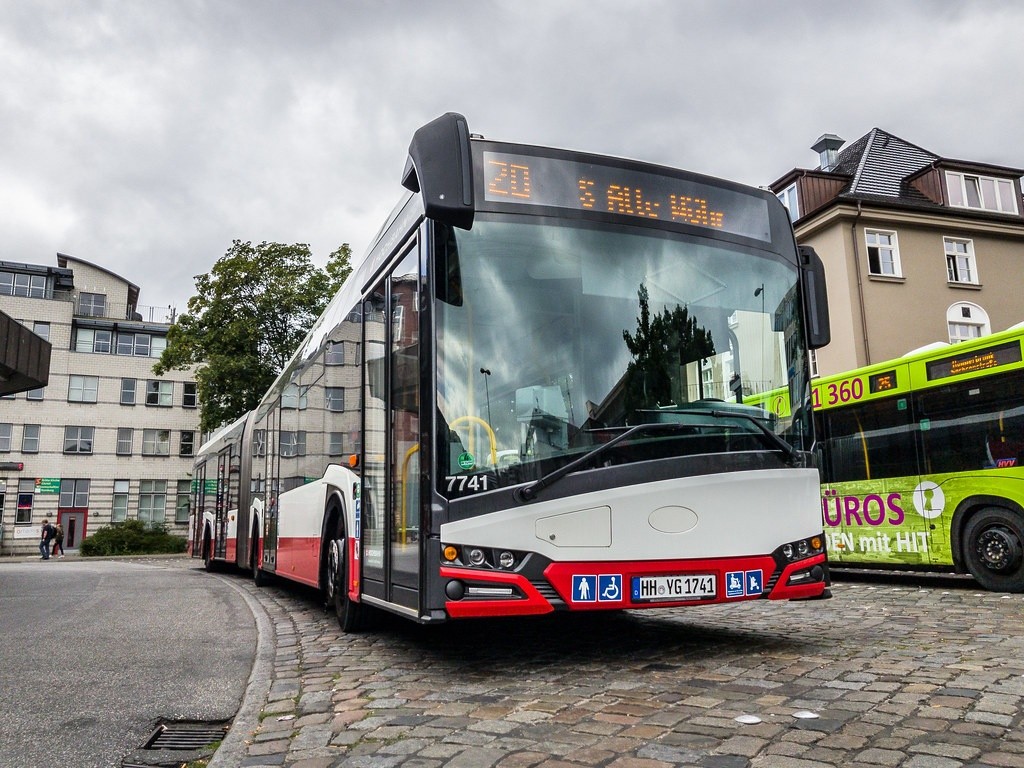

(187, 112), (833, 632)
(732, 320), (1023, 592)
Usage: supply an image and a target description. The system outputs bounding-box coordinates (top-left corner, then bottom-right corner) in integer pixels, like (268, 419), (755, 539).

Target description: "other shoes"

(40, 557), (49, 560)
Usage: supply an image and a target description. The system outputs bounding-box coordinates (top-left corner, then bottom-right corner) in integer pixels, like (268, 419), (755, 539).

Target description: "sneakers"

(48, 555), (54, 559)
(58, 555), (66, 559)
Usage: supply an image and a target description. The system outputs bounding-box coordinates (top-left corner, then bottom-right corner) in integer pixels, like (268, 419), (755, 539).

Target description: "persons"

(39, 519), (51, 560)
(49, 524), (65, 558)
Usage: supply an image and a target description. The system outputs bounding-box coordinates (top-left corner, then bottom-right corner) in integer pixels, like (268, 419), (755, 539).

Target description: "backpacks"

(51, 527), (57, 538)
